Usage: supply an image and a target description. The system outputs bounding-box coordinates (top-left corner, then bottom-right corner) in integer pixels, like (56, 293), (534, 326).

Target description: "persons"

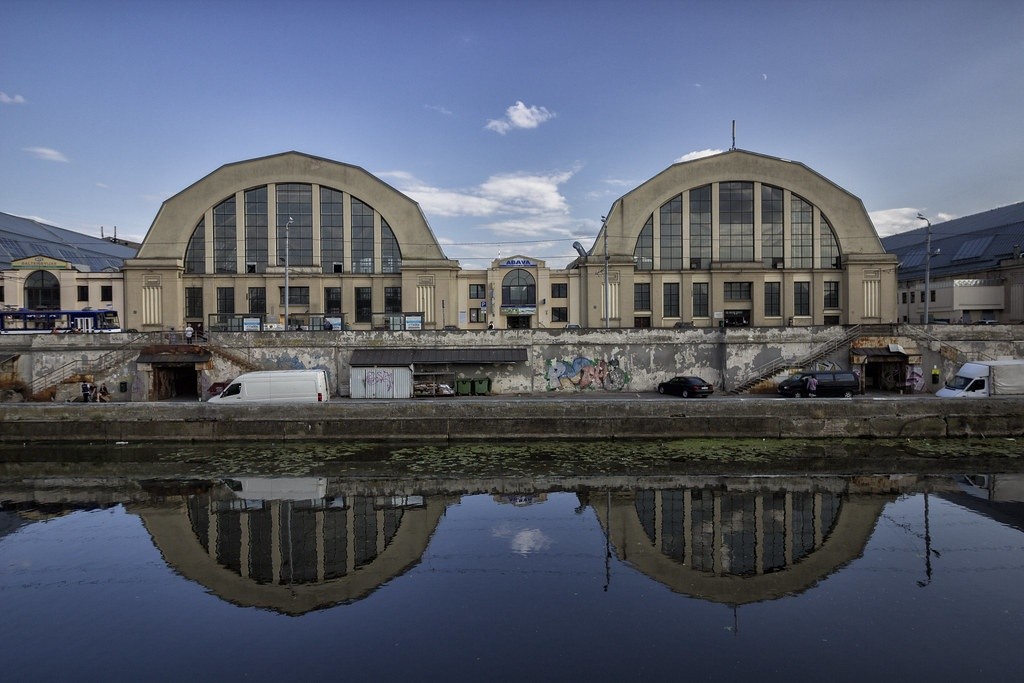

(82, 380), (109, 402)
(51, 329), (60, 333)
(488, 321), (494, 329)
(296, 325), (303, 330)
(185, 324), (194, 344)
(807, 375), (818, 398)
(958, 317), (963, 324)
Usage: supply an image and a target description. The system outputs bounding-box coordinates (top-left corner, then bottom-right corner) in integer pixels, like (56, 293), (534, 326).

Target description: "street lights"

(917, 216), (941, 324)
(286, 216), (295, 332)
(601, 214), (611, 328)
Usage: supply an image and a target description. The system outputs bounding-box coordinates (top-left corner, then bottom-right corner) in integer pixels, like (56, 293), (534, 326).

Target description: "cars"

(657, 376), (715, 398)
(563, 323), (582, 329)
(673, 322), (696, 328)
(443, 325), (460, 329)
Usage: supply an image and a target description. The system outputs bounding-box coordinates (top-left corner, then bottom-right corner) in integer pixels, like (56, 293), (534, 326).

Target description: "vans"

(217, 476), (328, 502)
(291, 316), (334, 331)
(206, 370), (330, 404)
(776, 370), (860, 399)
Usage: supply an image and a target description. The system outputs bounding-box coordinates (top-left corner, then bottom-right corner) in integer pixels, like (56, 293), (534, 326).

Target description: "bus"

(0, 307), (121, 335)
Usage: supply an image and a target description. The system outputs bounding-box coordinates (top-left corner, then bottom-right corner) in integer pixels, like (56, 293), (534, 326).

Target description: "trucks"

(936, 359), (1024, 398)
(958, 473), (1024, 504)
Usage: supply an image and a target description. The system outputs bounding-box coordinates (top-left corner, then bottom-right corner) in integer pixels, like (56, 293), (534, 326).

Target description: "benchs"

(86, 393), (109, 401)
